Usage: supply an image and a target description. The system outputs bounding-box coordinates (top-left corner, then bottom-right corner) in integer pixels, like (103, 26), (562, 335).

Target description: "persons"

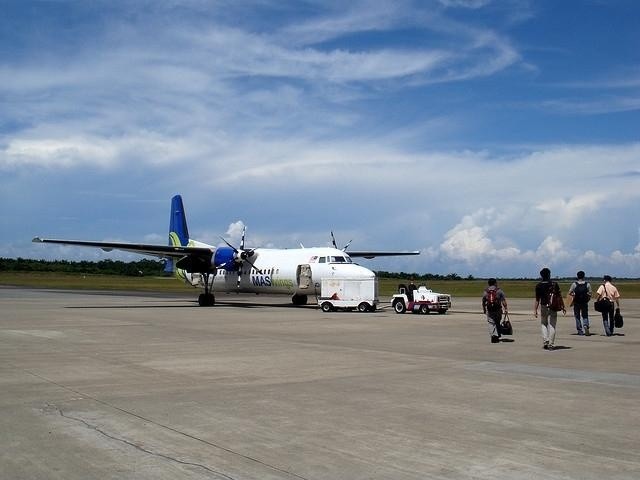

(482, 278), (507, 343)
(597, 275), (621, 336)
(569, 271), (593, 333)
(408, 280), (417, 293)
(534, 267), (567, 349)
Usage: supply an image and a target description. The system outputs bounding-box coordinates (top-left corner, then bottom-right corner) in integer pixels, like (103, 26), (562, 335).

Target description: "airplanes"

(30, 193), (420, 307)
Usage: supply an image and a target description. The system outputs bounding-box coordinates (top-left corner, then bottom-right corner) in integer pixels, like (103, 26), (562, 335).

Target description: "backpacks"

(485, 287), (500, 312)
(574, 281), (588, 305)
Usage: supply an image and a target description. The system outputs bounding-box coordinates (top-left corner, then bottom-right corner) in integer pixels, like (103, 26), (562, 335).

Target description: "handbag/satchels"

(594, 301), (601, 312)
(615, 307), (623, 328)
(600, 297), (612, 313)
(549, 282), (562, 311)
(500, 313), (513, 335)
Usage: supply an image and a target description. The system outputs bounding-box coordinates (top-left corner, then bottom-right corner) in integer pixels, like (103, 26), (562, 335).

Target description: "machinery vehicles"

(391, 282), (452, 314)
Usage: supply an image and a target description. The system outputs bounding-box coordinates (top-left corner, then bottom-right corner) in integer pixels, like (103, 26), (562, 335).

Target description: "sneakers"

(584, 325), (590, 335)
